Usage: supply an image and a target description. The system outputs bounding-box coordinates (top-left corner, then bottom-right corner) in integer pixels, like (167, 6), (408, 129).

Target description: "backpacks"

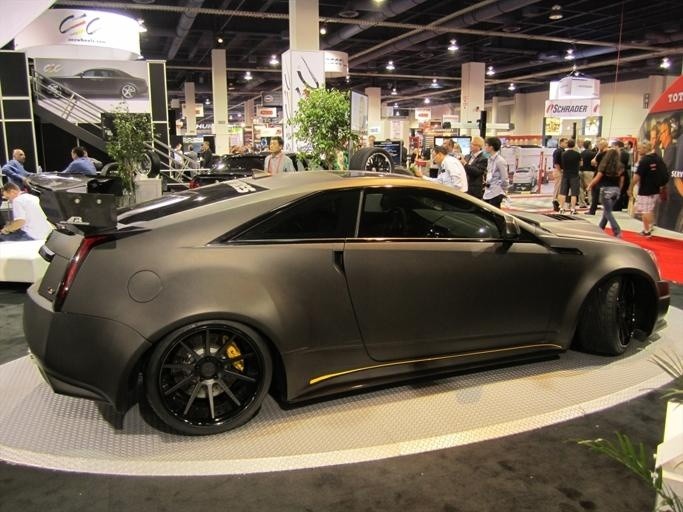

(645, 155), (669, 187)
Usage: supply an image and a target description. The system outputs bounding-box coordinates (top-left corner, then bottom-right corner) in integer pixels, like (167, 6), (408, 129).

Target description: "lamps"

(136, 11), (147, 34)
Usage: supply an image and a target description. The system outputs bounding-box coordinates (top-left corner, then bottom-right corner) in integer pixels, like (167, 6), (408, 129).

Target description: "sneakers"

(637, 224), (653, 238)
(559, 208), (578, 216)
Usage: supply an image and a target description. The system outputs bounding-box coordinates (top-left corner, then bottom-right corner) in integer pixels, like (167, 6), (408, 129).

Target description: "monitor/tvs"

(477, 111), (486, 139)
(433, 136), (472, 159)
(349, 88), (369, 137)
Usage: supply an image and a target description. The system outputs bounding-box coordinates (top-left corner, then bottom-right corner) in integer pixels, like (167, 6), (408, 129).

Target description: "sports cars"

(22, 168), (672, 436)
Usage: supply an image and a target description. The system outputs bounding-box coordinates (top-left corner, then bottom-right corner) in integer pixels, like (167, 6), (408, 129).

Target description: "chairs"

(0, 165), (14, 223)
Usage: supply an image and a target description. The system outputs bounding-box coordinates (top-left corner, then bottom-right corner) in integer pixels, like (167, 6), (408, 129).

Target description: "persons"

(262, 136), (293, 173)
(650, 126), (661, 156)
(658, 119), (675, 227)
(459, 135), (486, 198)
(670, 135), (683, 233)
(229, 144), (253, 155)
(416, 146), (468, 194)
(368, 135), (376, 148)
(550, 135), (669, 240)
(482, 136), (509, 208)
(440, 139), (456, 157)
(56, 146), (96, 178)
(452, 143), (462, 155)
(81, 147), (103, 170)
(170, 141), (214, 184)
(0, 149), (35, 191)
(0, 182), (57, 240)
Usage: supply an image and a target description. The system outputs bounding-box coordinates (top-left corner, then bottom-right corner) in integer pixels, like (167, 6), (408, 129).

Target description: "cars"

(36, 68), (148, 100)
(189, 152), (333, 189)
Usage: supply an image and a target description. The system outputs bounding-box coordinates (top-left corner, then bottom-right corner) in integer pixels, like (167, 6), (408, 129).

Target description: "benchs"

(0, 240), (57, 286)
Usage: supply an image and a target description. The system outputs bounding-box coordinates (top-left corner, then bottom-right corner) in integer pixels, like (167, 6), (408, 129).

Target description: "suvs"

(512, 165), (538, 191)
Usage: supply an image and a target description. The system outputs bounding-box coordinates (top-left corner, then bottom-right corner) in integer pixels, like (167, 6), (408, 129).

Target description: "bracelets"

(462, 162), (466, 167)
